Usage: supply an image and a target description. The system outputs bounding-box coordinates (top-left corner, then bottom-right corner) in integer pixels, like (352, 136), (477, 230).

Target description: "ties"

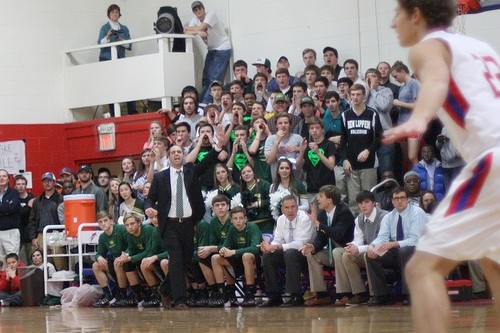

(176, 170), (183, 219)
(288, 221), (294, 242)
(328, 216), (333, 265)
(396, 214), (404, 241)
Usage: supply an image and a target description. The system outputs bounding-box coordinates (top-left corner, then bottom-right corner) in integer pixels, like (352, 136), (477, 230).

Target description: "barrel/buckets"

(64, 195), (95, 240)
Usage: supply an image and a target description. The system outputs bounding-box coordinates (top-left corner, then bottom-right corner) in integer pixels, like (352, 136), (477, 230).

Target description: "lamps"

(98, 122), (116, 134)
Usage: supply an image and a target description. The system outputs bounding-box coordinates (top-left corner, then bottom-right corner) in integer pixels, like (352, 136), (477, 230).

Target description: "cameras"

(111, 29), (124, 35)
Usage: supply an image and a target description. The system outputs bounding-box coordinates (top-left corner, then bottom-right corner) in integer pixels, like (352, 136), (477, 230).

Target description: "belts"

(167, 218), (193, 222)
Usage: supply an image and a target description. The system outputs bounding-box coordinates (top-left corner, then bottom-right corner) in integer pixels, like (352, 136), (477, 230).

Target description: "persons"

(382, 0), (500, 333)
(0, 47), (492, 308)
(184, 1), (231, 104)
(97, 4), (139, 117)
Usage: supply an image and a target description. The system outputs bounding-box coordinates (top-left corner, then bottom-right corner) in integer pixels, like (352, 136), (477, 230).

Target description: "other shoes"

(90, 287), (409, 310)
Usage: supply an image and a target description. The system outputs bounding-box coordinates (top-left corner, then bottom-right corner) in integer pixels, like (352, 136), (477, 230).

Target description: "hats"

(252, 57), (271, 67)
(323, 46), (338, 57)
(271, 92), (287, 104)
(41, 172), (56, 181)
(77, 164), (92, 172)
(59, 167), (72, 176)
(299, 96), (314, 105)
(191, 0), (205, 10)
